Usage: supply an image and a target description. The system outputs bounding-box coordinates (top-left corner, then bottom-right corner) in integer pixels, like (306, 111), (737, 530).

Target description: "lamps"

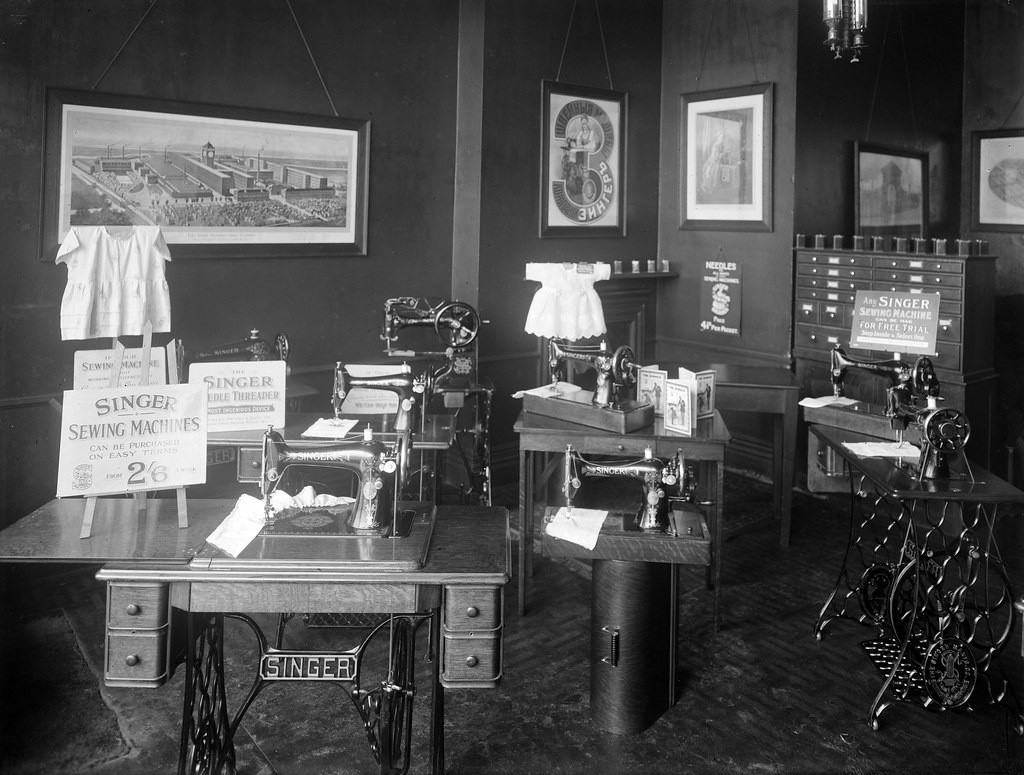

(822, 0), (868, 64)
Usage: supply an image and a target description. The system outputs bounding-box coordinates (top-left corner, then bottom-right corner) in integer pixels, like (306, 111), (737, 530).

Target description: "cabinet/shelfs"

(792, 246), (1000, 540)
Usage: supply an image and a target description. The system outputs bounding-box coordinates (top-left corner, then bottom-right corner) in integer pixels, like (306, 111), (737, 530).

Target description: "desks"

(619, 360), (799, 550)
(513, 409), (732, 633)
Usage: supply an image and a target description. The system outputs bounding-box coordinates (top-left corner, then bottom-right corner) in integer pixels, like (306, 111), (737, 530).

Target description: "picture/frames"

(970, 127), (1024, 234)
(537, 77), (629, 243)
(677, 77), (778, 230)
(851, 138), (931, 236)
(40, 88), (371, 259)
